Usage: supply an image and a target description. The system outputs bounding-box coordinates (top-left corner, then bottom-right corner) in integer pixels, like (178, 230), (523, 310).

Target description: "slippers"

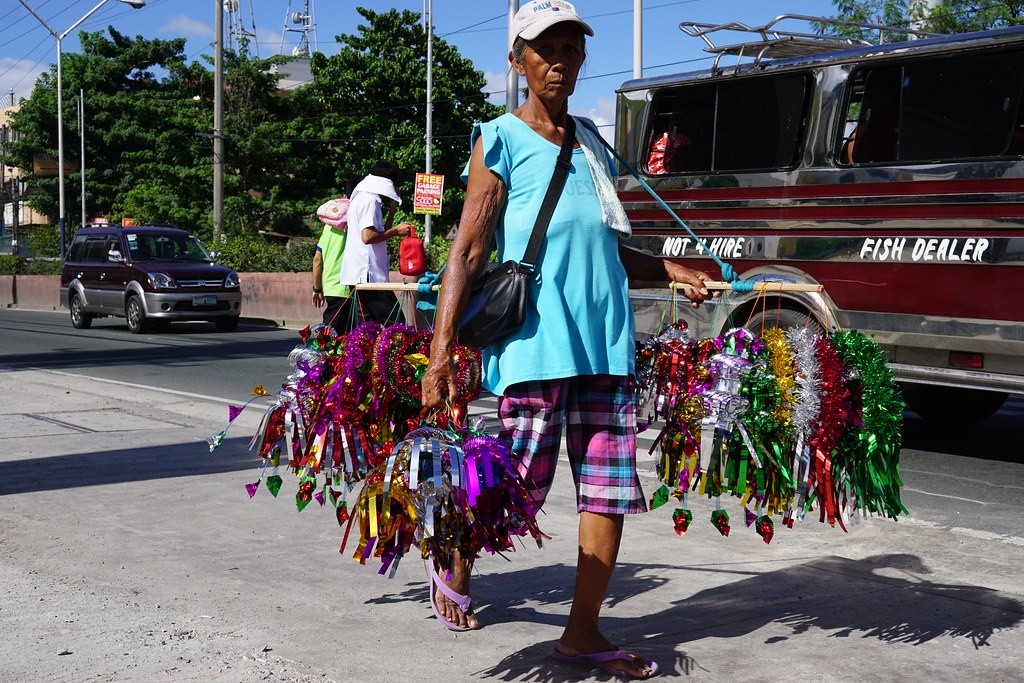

(428, 558), (481, 632)
(552, 641), (660, 680)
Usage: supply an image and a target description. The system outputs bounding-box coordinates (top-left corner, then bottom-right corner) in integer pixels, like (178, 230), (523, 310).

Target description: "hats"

(508, 1), (594, 49)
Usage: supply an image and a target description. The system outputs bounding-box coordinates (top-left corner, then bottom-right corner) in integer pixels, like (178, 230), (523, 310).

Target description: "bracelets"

(313, 286), (322, 293)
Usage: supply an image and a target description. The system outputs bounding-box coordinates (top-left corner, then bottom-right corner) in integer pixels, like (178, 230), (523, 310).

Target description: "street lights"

(17, 0), (146, 258)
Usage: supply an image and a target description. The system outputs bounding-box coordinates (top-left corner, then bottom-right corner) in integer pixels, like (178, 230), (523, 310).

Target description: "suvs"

(59, 222), (242, 333)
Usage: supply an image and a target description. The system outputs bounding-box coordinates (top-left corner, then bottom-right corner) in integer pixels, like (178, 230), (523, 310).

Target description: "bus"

(612, 13), (1024, 432)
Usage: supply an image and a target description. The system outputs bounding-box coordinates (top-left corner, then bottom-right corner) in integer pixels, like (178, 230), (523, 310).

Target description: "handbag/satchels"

(456, 253), (532, 347)
(399, 225), (426, 275)
(316, 197), (349, 231)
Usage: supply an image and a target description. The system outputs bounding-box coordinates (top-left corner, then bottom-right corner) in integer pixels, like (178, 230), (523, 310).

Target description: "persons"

(340, 160), (411, 326)
(421, 0), (723, 678)
(311, 175), (366, 335)
